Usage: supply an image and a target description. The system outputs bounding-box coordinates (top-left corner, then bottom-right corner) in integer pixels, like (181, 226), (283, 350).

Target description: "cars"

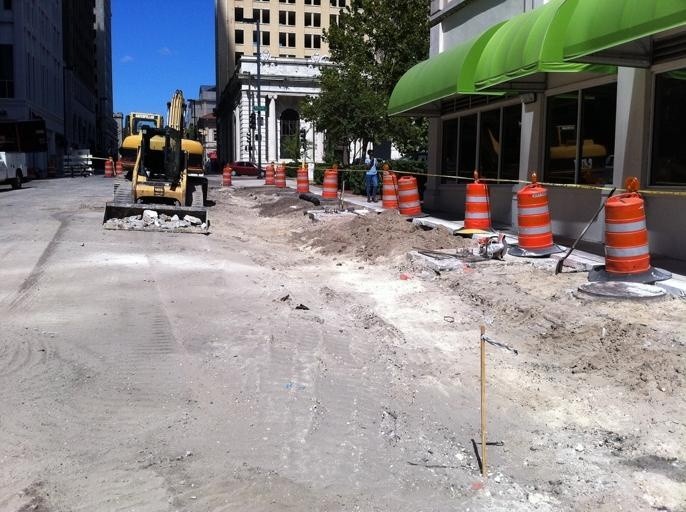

(230, 161), (267, 178)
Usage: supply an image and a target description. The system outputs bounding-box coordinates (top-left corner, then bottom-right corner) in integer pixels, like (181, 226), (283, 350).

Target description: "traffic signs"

(253, 106), (266, 111)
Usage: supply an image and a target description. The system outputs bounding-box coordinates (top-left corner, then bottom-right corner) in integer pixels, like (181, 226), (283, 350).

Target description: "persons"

(365, 149), (379, 203)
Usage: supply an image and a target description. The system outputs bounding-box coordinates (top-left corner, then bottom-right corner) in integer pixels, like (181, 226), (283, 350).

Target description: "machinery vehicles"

(101, 90), (209, 233)
(545, 124), (608, 182)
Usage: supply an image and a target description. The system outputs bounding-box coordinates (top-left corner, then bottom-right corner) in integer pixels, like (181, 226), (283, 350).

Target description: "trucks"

(0, 152), (27, 189)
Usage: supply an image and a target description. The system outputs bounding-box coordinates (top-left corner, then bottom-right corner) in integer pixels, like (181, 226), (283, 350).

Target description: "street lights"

(242, 70), (251, 160)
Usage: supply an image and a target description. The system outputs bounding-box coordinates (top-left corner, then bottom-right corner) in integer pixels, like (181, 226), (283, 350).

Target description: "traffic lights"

(246, 132), (251, 143)
(249, 113), (256, 130)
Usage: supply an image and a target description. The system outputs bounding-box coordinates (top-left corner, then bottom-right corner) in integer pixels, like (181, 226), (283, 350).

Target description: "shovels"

(555, 187), (617, 275)
